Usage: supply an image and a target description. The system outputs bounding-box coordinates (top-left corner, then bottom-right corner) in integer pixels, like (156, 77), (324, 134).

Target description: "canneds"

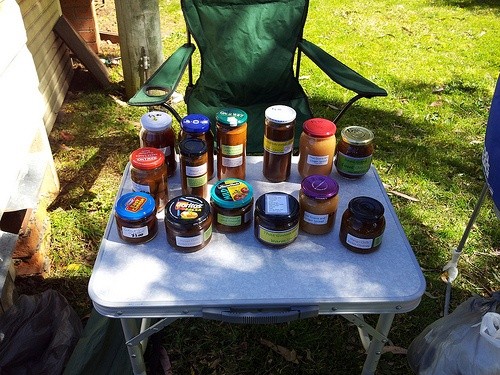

(139, 111), (177, 179)
(299, 176), (339, 234)
(215, 107), (246, 182)
(335, 125), (374, 180)
(339, 196), (386, 253)
(263, 105), (296, 182)
(129, 147), (169, 212)
(179, 113), (214, 181)
(298, 118), (336, 178)
(165, 195), (212, 252)
(253, 191), (300, 247)
(180, 137), (208, 200)
(210, 177), (253, 233)
(114, 191), (158, 245)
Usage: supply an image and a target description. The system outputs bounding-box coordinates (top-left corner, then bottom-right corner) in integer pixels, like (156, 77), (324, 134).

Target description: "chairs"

(443, 73), (500, 317)
(128, 1), (388, 157)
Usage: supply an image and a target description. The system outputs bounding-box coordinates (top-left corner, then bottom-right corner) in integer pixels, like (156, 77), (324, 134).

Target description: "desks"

(88, 157), (426, 375)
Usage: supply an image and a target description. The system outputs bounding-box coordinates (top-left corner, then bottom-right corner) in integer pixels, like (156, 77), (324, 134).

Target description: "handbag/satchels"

(407, 291), (500, 375)
